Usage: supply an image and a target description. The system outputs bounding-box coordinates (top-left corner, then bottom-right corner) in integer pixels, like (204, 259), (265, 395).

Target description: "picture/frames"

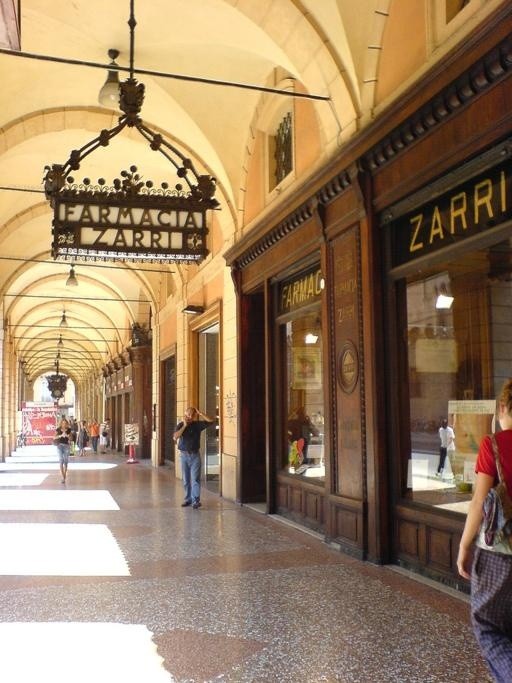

(291, 346), (323, 390)
(408, 325), (459, 383)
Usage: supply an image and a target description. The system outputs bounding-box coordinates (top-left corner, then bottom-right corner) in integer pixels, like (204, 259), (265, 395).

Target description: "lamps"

(62, 264), (78, 290)
(97, 49), (124, 109)
(181, 306), (204, 315)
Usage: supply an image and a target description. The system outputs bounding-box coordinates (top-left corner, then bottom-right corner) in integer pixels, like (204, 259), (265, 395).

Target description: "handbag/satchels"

(102, 432), (107, 436)
(475, 483), (512, 556)
(84, 436), (89, 441)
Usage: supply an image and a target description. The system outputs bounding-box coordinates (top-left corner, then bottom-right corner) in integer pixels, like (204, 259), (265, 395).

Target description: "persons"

(173, 407), (214, 509)
(59, 415), (111, 457)
(288, 405), (319, 469)
(53, 419), (73, 484)
(435, 419), (456, 476)
(456, 379), (512, 683)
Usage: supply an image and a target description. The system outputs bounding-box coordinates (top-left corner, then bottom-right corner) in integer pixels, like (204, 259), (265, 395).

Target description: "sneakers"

(193, 503), (201, 508)
(182, 502), (191, 506)
